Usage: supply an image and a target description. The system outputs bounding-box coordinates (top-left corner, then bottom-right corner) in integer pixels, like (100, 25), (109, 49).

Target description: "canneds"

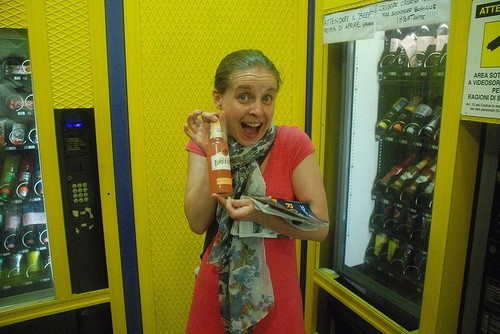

(9, 251), (41, 277)
(372, 199), (434, 272)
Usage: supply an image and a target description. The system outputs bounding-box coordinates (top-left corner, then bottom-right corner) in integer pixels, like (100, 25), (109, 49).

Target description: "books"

(230, 195), (329, 238)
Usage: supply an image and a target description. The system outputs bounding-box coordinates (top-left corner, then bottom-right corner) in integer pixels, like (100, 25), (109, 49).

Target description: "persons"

(183, 48), (329, 334)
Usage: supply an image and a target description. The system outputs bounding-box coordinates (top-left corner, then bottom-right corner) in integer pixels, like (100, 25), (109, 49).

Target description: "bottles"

(365, 24), (449, 279)
(0, 151), (51, 282)
(207, 112), (232, 197)
